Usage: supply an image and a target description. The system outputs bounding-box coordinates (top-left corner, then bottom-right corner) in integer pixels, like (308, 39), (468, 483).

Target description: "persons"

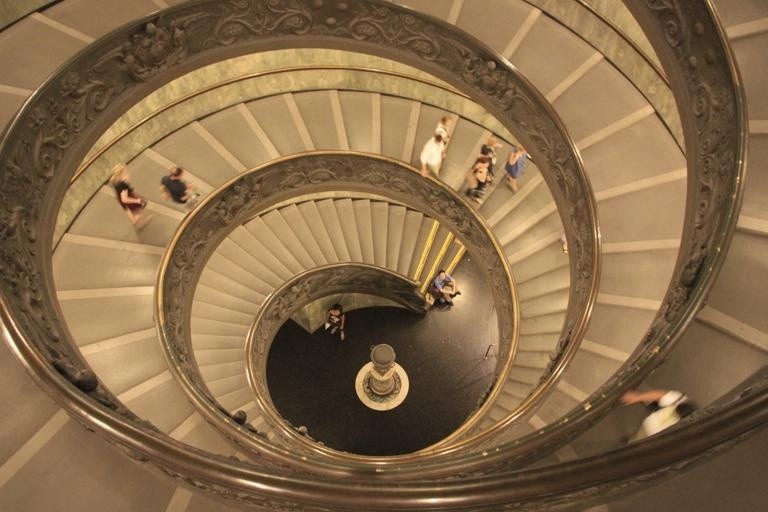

(467, 156), (489, 206)
(619, 389), (701, 449)
(158, 163), (200, 210)
(435, 117), (453, 144)
(108, 162), (151, 232)
(487, 136), (504, 178)
(325, 301), (346, 337)
(505, 145), (527, 193)
(480, 145), (494, 185)
(433, 267), (462, 306)
(420, 132), (447, 178)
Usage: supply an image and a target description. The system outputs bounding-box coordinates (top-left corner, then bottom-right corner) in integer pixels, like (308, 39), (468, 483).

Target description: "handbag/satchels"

(647, 402), (660, 411)
